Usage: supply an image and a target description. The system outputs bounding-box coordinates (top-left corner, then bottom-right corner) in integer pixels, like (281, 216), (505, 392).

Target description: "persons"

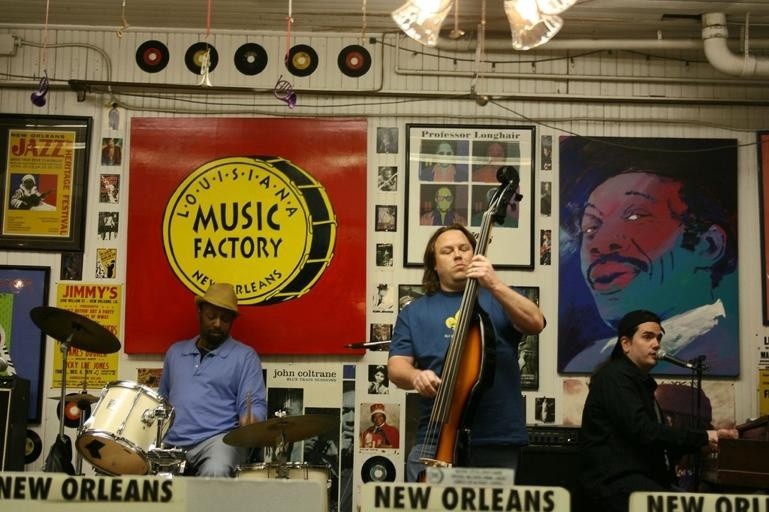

(365, 125), (400, 451)
(269, 392), (302, 463)
(342, 381), (354, 465)
(155, 282), (268, 480)
(303, 434), (339, 468)
(472, 141), (509, 182)
(577, 308), (741, 492)
(9, 173), (48, 211)
(420, 141), (468, 182)
(559, 157), (737, 374)
(540, 398), (554, 422)
(386, 224), (548, 484)
(100, 136), (121, 278)
(420, 184), (468, 226)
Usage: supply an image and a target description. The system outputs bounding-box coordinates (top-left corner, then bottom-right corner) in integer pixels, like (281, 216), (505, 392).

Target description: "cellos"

(419, 164), (522, 480)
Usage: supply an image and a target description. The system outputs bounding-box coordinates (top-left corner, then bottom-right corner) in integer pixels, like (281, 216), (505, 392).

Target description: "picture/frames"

(403, 123), (536, 271)
(0, 113), (94, 253)
(756, 130), (769, 325)
(1, 265), (52, 427)
(398, 285), (539, 388)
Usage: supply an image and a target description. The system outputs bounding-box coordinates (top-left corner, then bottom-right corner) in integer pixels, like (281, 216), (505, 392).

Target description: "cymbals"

(28, 306), (121, 354)
(221, 413), (338, 449)
(46, 393), (99, 404)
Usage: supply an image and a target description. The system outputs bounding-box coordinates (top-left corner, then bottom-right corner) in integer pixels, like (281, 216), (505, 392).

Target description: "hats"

(369, 403), (386, 422)
(195, 282), (241, 315)
(21, 174), (36, 186)
(617, 310), (661, 339)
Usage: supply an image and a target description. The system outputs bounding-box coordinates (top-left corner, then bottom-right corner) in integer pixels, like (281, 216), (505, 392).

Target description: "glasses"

(436, 195), (454, 201)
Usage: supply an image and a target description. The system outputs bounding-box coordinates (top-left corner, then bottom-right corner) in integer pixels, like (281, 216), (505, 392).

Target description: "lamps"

(390, 0), (576, 50)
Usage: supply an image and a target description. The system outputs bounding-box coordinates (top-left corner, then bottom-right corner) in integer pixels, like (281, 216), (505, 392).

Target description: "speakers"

(515, 425), (593, 512)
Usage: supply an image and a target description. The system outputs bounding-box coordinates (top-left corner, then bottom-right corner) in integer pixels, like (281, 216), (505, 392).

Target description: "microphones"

(657, 349), (691, 371)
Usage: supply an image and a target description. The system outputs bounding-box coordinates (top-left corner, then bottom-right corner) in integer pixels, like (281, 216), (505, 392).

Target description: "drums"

(161, 155), (338, 308)
(75, 379), (174, 476)
(231, 459), (333, 486)
